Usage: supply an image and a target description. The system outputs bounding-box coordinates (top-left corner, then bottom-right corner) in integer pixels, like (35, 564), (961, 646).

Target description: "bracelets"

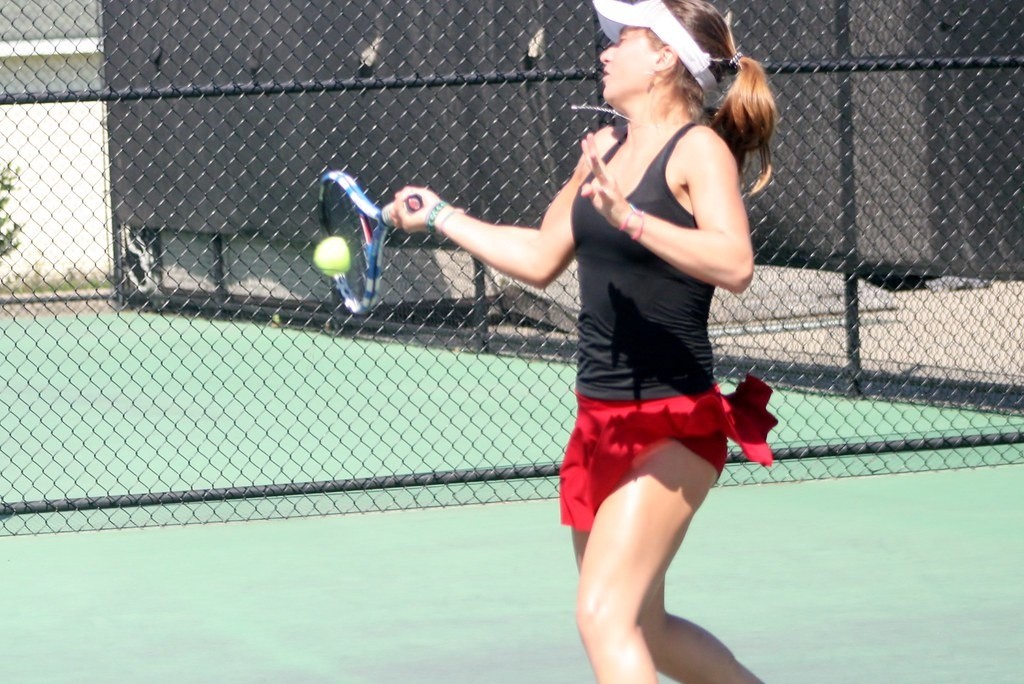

(440, 208), (463, 237)
(618, 201), (646, 241)
(425, 199), (447, 235)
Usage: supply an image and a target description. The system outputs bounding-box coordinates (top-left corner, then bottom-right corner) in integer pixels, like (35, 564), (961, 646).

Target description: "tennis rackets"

(315, 168), (424, 316)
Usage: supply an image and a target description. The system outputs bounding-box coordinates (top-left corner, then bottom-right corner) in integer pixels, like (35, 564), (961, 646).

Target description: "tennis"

(312, 236), (352, 277)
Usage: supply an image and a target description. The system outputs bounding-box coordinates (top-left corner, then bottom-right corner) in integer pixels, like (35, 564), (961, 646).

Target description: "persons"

(388, 0), (780, 684)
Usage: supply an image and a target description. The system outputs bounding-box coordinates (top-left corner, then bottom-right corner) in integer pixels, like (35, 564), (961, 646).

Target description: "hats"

(593, 2), (719, 97)
